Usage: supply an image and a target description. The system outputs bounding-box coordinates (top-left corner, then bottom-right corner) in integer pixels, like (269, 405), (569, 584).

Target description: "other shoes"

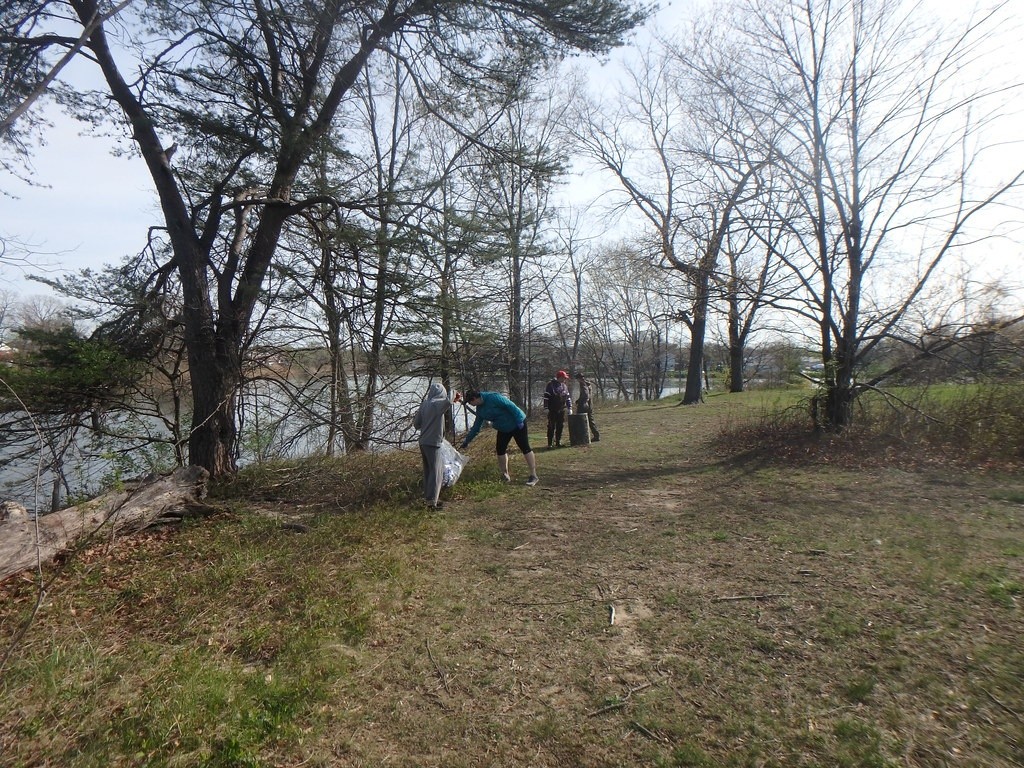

(427, 504), (444, 511)
(526, 474), (539, 486)
(437, 501), (443, 506)
(498, 472), (510, 483)
(591, 437), (600, 442)
(556, 440), (562, 446)
(547, 445), (554, 450)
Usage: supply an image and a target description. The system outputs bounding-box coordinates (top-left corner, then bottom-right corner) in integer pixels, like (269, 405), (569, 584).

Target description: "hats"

(556, 370), (569, 379)
(575, 373), (584, 379)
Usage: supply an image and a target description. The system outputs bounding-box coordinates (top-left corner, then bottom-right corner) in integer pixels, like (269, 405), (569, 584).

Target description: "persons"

(413, 383), (461, 512)
(543, 371), (573, 448)
(458, 389), (539, 486)
(574, 373), (601, 442)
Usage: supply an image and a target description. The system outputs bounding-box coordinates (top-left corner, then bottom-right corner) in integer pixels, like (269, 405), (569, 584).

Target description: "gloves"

(567, 409), (573, 415)
(544, 409), (550, 414)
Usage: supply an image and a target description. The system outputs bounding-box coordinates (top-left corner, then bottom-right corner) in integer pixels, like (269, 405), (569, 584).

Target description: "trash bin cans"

(567, 412), (592, 446)
